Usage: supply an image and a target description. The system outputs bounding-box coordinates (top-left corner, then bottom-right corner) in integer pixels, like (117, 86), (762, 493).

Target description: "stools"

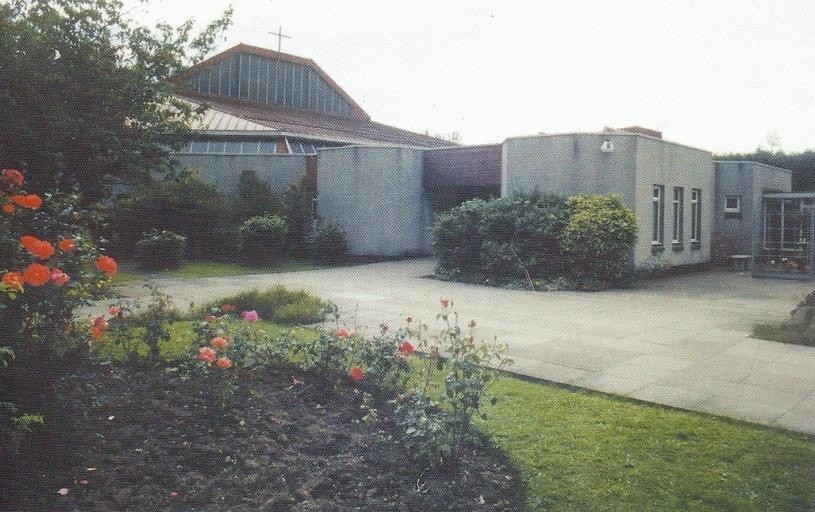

(730, 254), (752, 271)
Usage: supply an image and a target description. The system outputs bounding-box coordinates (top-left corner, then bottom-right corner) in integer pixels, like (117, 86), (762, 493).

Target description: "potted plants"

(235, 210), (348, 266)
(137, 225), (186, 272)
